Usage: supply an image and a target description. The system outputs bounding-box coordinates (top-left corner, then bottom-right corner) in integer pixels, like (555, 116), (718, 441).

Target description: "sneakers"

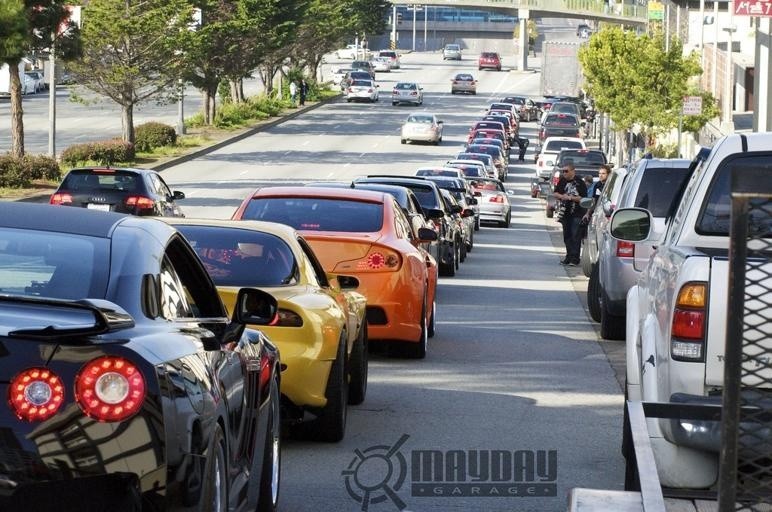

(568, 261), (579, 267)
(559, 260), (569, 266)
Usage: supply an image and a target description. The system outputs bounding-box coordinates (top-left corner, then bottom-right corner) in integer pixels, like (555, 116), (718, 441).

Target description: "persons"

(509, 133), (530, 163)
(636, 127), (646, 159)
(583, 174), (595, 198)
(552, 164), (588, 268)
(624, 127), (637, 164)
(298, 79), (310, 106)
(581, 165), (611, 222)
(584, 106), (598, 138)
(287, 79), (301, 108)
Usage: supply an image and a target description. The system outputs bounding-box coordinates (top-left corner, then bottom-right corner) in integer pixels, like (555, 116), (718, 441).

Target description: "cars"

(442, 43), (463, 60)
(335, 45), (370, 59)
(341, 71), (371, 90)
(1, 202), (282, 512)
(443, 164), (486, 179)
(303, 181), (440, 270)
(333, 67), (353, 85)
(346, 80), (379, 103)
(230, 186), (438, 359)
(440, 189), (474, 263)
(352, 60), (376, 81)
(141, 215), (368, 441)
(478, 51), (503, 71)
(450, 73), (478, 95)
(379, 51), (402, 68)
(401, 112), (444, 146)
(372, 57), (390, 72)
(447, 160), (489, 178)
(392, 82), (423, 107)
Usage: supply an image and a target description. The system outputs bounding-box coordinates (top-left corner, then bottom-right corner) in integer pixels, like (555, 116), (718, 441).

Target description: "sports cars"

(464, 176), (514, 227)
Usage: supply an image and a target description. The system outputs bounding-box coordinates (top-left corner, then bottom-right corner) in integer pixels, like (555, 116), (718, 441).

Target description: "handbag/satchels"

(573, 218), (590, 241)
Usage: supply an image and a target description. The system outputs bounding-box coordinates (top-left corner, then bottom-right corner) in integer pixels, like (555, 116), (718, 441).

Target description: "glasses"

(561, 168), (574, 174)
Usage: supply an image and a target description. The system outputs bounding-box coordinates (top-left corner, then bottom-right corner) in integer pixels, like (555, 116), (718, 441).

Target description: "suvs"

(610, 132), (772, 492)
(456, 178), (482, 230)
(355, 174), (463, 277)
(465, 144), (508, 181)
(483, 115), (515, 145)
(415, 167), (465, 178)
(471, 138), (511, 165)
(546, 149), (614, 217)
(474, 120), (505, 133)
(456, 152), (498, 180)
(490, 103), (521, 129)
(487, 109), (517, 130)
(536, 137), (587, 183)
(501, 96), (538, 121)
(549, 102), (581, 125)
(538, 112), (586, 144)
(587, 156), (694, 339)
(581, 164), (627, 275)
(473, 129), (506, 146)
(48, 167), (186, 217)
(420, 176), (478, 252)
